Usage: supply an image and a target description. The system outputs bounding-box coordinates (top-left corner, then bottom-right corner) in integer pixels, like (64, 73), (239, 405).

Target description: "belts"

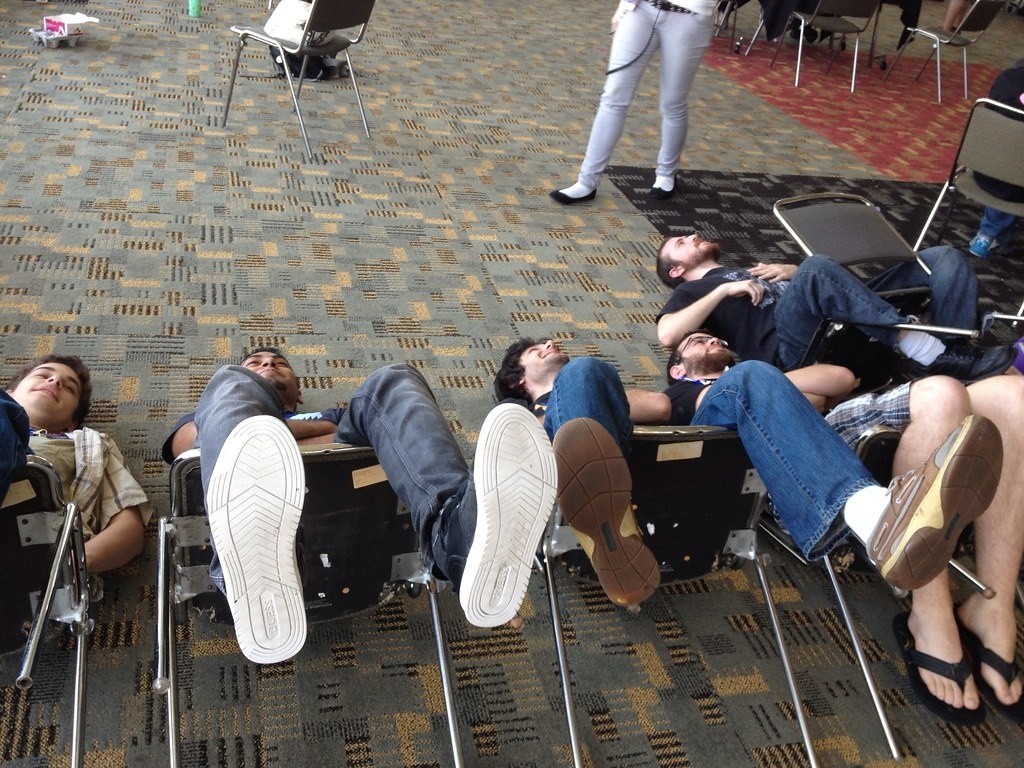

(647, 0), (697, 15)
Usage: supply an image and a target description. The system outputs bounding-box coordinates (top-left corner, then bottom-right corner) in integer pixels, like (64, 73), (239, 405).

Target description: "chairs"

(714, 0), (1005, 105)
(773, 97), (1024, 758)
(154, 441), (464, 768)
(0, 456), (93, 768)
(223, 0), (376, 159)
(535, 424), (818, 768)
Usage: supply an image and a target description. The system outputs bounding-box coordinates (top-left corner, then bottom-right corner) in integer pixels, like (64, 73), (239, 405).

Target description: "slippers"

(953, 606), (1024, 719)
(893, 610), (986, 726)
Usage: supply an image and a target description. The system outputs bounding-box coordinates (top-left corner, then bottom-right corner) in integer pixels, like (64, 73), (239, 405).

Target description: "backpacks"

(270, 45), (348, 81)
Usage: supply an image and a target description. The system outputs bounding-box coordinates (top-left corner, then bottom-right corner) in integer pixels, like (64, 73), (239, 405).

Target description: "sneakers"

(208, 414), (308, 664)
(554, 418), (660, 606)
(431, 403), (558, 627)
(864, 414), (1004, 590)
(969, 232), (1014, 259)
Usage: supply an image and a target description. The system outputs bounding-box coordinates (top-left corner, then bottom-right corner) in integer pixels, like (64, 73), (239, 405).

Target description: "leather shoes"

(904, 342), (1015, 382)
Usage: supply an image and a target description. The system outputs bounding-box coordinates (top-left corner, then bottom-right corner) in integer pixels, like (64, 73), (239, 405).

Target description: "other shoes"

(650, 175), (676, 198)
(549, 188), (597, 204)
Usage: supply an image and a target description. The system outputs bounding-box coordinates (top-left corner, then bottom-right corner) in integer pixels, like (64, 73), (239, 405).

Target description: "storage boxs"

(43, 12), (99, 38)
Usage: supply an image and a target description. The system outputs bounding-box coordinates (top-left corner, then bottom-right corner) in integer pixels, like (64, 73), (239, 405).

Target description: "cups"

(188, 0), (201, 17)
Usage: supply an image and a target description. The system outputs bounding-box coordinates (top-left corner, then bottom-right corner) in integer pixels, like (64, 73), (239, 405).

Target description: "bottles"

(879, 55), (887, 70)
(734, 36), (743, 54)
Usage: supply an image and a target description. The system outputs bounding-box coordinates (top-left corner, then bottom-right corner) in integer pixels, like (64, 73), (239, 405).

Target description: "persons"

(968, 57), (1024, 260)
(548, 0), (721, 205)
(943, 0), (976, 38)
(0, 356), (149, 655)
(496, 335), (1002, 608)
(656, 231), (1024, 723)
(162, 348), (558, 664)
